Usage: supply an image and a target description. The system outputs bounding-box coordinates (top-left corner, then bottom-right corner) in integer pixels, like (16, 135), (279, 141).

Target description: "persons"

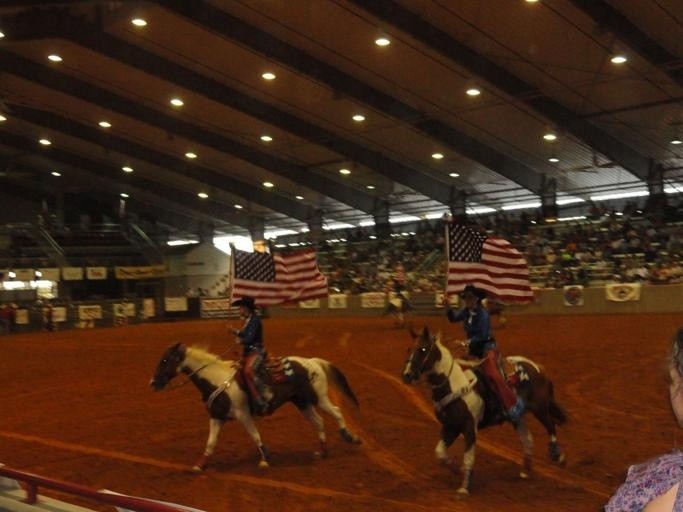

(197, 191), (682, 297)
(440, 282), (531, 438)
(222, 294), (271, 415)
(599, 325), (683, 511)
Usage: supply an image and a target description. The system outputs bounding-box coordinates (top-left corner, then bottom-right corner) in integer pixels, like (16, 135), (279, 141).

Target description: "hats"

(230, 296), (261, 310)
(457, 286), (489, 300)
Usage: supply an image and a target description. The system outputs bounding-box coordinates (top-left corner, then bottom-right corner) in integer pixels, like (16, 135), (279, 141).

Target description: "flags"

(445, 223), (536, 302)
(230, 245), (330, 308)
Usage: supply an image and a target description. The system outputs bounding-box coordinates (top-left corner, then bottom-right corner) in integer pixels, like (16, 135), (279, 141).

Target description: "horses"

(400, 321), (570, 500)
(148, 339), (362, 476)
